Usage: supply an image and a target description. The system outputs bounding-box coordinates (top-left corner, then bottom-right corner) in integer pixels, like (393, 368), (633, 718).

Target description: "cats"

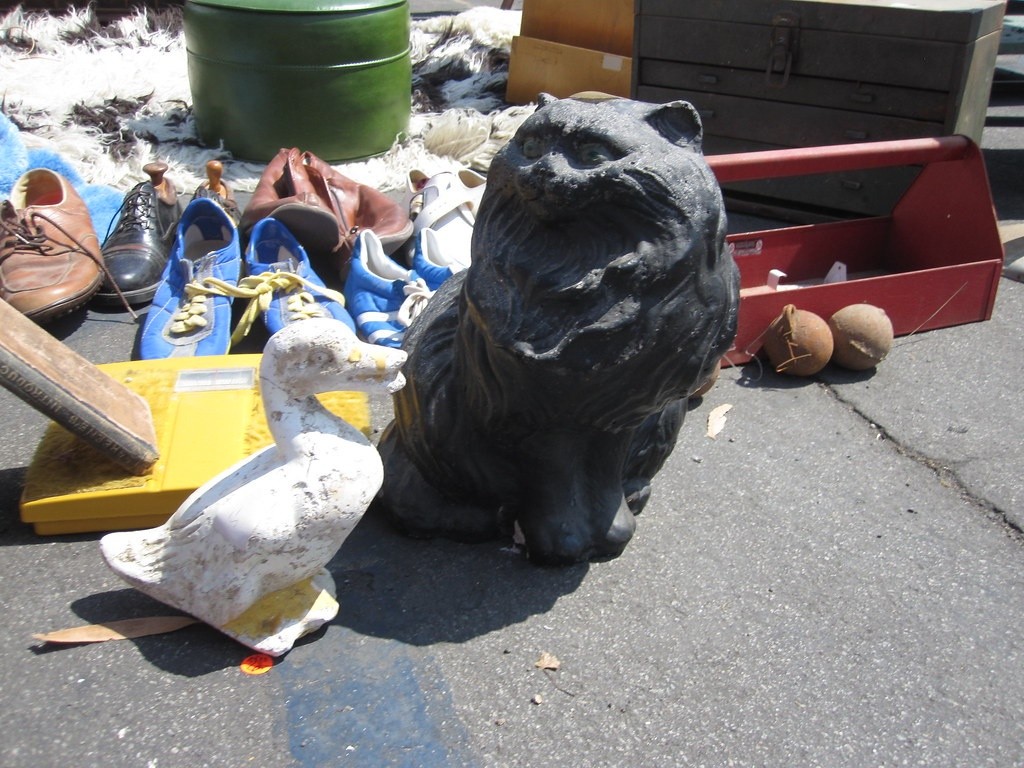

(373, 92), (742, 568)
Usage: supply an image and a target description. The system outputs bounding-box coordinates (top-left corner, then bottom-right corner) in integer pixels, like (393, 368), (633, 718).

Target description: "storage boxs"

(629, 0), (1008, 226)
(503, 1), (636, 104)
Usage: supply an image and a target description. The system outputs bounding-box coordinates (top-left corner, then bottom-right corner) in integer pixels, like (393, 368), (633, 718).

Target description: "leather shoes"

(0, 166), (415, 321)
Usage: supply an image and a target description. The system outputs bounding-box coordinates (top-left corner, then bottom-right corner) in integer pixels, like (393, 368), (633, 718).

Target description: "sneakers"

(343, 227), (469, 350)
(140, 198), (355, 362)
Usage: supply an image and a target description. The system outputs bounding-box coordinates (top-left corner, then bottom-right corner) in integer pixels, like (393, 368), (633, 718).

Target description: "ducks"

(95, 318), (409, 657)
